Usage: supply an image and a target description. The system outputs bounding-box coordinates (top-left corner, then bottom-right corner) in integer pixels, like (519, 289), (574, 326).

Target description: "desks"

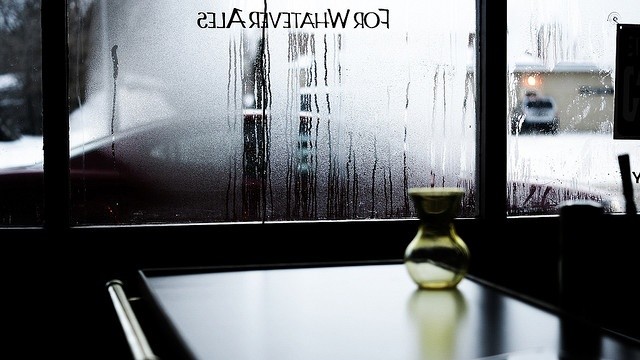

(104, 260), (640, 359)
